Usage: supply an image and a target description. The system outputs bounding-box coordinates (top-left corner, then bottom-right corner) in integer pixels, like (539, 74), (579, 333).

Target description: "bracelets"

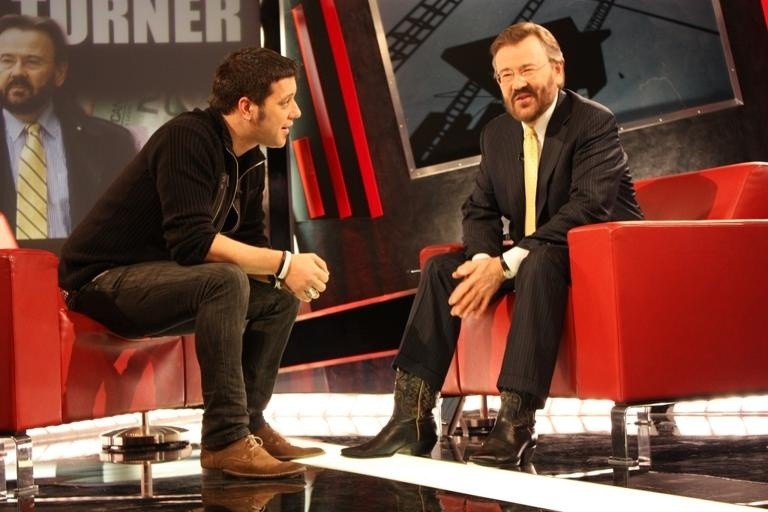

(274, 277), (281, 290)
(274, 250), (291, 279)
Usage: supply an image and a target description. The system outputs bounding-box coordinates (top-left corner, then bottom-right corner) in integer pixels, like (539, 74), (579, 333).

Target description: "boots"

(341, 365), (439, 457)
(470, 383), (539, 467)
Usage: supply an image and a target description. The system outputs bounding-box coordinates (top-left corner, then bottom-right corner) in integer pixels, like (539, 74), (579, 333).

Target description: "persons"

(0, 14), (136, 241)
(336, 22), (646, 469)
(57, 49), (325, 477)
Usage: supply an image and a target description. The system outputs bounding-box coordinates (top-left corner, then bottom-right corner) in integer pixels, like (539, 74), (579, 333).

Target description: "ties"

(14, 123), (50, 240)
(522, 123), (539, 238)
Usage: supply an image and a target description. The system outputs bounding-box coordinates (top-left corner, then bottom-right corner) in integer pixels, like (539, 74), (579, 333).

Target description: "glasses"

(495, 60), (553, 86)
(1, 53), (64, 69)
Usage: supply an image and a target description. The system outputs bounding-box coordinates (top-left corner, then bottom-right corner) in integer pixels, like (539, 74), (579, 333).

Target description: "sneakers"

(250, 421), (326, 460)
(199, 435), (308, 480)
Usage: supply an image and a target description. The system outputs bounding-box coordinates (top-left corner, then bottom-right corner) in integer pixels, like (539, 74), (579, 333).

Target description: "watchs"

(500, 254), (515, 279)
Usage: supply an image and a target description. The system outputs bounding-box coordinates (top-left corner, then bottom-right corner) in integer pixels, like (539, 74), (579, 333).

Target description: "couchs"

(419, 161), (768, 488)
(2, 248), (205, 509)
